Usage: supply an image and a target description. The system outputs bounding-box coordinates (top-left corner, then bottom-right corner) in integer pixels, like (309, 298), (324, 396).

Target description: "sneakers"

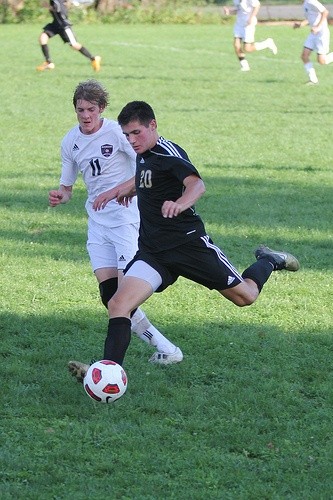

(148, 346), (184, 365)
(266, 38), (278, 55)
(91, 56), (101, 72)
(240, 60), (250, 71)
(67, 359), (96, 383)
(255, 247), (300, 271)
(36, 62), (55, 71)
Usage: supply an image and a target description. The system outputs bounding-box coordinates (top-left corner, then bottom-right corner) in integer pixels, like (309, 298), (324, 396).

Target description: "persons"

(49, 81), (183, 365)
(292, 0), (333, 85)
(224, 0), (277, 71)
(36, 0), (101, 73)
(68, 101), (300, 385)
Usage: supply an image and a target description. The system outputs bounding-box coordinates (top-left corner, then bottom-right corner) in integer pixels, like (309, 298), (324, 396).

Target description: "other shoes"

(305, 80), (319, 86)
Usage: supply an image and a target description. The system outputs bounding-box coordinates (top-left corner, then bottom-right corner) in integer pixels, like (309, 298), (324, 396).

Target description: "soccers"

(82, 360), (128, 402)
